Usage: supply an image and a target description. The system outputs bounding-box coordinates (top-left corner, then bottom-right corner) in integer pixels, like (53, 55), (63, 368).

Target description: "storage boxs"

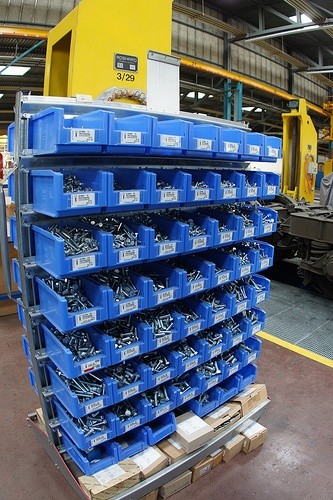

(8, 107), (284, 500)
